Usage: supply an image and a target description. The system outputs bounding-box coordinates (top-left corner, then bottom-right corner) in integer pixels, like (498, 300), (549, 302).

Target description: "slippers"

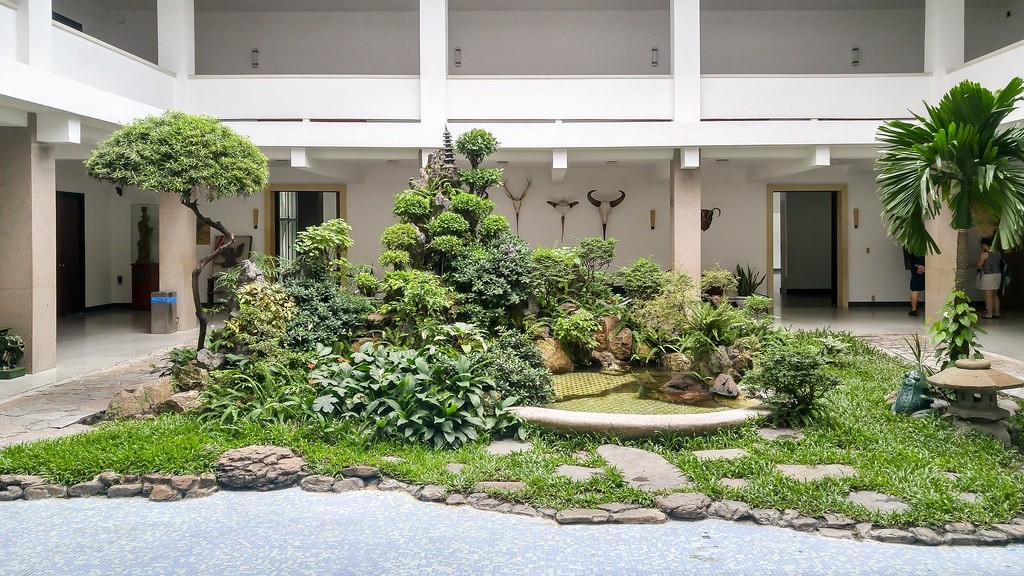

(992, 314), (1000, 318)
(982, 315), (993, 319)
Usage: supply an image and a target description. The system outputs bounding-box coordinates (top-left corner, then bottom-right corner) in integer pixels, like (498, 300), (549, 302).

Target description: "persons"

(900, 221), (925, 317)
(975, 239), (1008, 319)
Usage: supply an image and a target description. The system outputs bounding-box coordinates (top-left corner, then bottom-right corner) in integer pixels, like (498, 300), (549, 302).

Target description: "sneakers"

(908, 310), (917, 316)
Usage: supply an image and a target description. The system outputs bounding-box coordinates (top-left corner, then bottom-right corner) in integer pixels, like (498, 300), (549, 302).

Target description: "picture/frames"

(210, 235), (253, 275)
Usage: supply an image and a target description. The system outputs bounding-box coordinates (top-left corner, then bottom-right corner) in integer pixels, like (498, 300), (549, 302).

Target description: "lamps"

(652, 49), (657, 67)
(252, 48), (258, 68)
(852, 47), (859, 66)
(455, 48), (461, 67)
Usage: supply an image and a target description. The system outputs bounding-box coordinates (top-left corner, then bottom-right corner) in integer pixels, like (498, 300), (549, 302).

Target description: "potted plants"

(725, 258), (766, 309)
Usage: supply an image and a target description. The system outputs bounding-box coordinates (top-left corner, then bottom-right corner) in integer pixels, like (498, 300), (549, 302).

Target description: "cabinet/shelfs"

(130, 263), (159, 312)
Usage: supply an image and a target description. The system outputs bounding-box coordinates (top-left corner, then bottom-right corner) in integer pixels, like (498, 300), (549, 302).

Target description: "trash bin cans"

(150, 290), (176, 335)
